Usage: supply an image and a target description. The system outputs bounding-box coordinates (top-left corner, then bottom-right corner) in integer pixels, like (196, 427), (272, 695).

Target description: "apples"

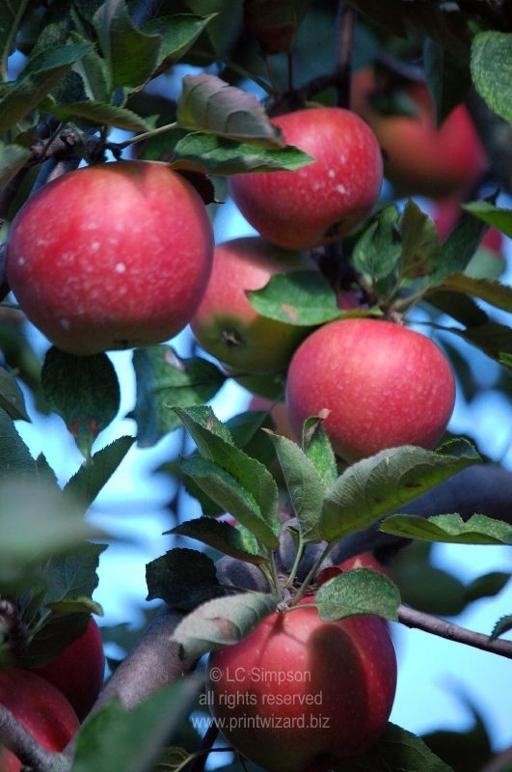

(2, 64), (503, 772)
